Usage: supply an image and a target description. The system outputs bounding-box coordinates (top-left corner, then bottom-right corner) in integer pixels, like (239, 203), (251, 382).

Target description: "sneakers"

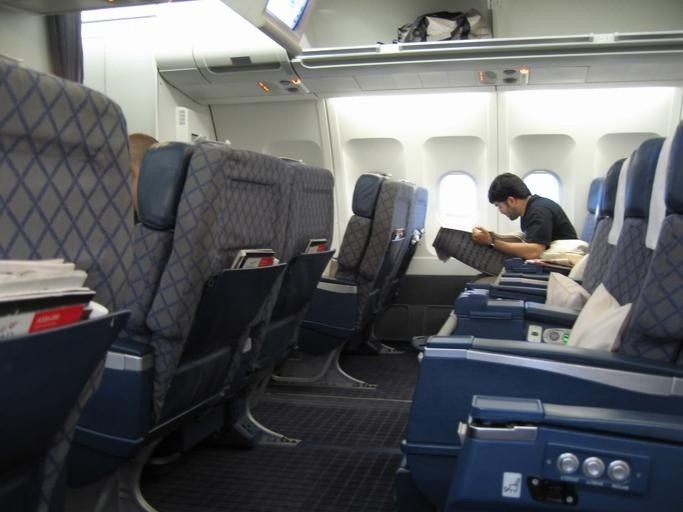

(410, 336), (429, 350)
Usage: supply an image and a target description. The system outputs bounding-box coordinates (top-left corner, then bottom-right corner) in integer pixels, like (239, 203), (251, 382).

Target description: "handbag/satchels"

(397, 10), (470, 43)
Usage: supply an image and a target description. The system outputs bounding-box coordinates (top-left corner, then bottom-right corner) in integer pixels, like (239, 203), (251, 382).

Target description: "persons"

(128, 133), (159, 214)
(471, 172), (579, 283)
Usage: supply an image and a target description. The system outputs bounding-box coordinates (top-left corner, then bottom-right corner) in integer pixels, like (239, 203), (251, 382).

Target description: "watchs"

(489, 237), (496, 249)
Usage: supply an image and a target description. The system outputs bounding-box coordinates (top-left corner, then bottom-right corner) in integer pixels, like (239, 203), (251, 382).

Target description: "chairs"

(0, 50), (431, 511)
(399, 119), (682, 512)
